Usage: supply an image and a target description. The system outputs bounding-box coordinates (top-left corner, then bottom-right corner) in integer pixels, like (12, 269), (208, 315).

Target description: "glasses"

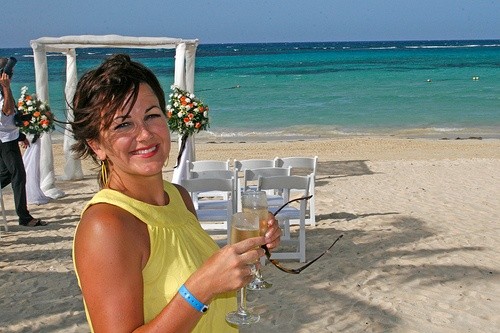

(261, 195), (343, 274)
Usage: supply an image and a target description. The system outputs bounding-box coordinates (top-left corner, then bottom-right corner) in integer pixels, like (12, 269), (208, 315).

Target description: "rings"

(248, 264), (257, 275)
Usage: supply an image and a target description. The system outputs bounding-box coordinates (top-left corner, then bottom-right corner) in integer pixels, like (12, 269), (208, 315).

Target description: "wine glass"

(225, 211), (261, 325)
(244, 191), (273, 291)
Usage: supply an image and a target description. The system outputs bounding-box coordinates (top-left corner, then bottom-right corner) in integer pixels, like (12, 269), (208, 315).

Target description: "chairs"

(180, 155), (318, 264)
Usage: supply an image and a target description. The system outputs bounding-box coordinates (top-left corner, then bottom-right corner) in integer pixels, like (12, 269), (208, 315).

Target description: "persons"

(49, 53), (283, 333)
(0, 58), (49, 226)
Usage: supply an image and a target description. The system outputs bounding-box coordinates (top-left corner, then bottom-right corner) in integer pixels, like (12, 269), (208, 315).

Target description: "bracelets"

(177, 284), (208, 313)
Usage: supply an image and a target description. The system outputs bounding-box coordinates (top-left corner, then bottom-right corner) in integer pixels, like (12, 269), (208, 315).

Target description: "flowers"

(15, 85), (56, 144)
(166, 83), (211, 137)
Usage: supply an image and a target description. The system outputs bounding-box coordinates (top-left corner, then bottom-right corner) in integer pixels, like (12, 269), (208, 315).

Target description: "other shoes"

(26, 218), (42, 227)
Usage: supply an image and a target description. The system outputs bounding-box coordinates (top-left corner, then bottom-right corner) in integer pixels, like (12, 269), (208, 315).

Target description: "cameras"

(0, 57), (17, 78)
